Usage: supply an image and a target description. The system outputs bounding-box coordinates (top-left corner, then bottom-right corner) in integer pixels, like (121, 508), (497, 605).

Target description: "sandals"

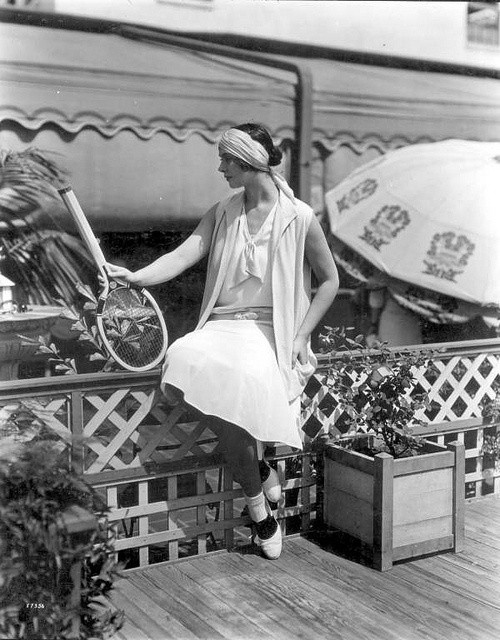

(257, 499), (283, 559)
(263, 457), (281, 502)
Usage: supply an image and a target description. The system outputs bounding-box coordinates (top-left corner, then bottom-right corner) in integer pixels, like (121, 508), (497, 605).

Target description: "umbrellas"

(323, 139), (500, 308)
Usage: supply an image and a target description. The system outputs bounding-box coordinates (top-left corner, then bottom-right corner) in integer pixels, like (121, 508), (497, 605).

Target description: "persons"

(99, 124), (343, 560)
